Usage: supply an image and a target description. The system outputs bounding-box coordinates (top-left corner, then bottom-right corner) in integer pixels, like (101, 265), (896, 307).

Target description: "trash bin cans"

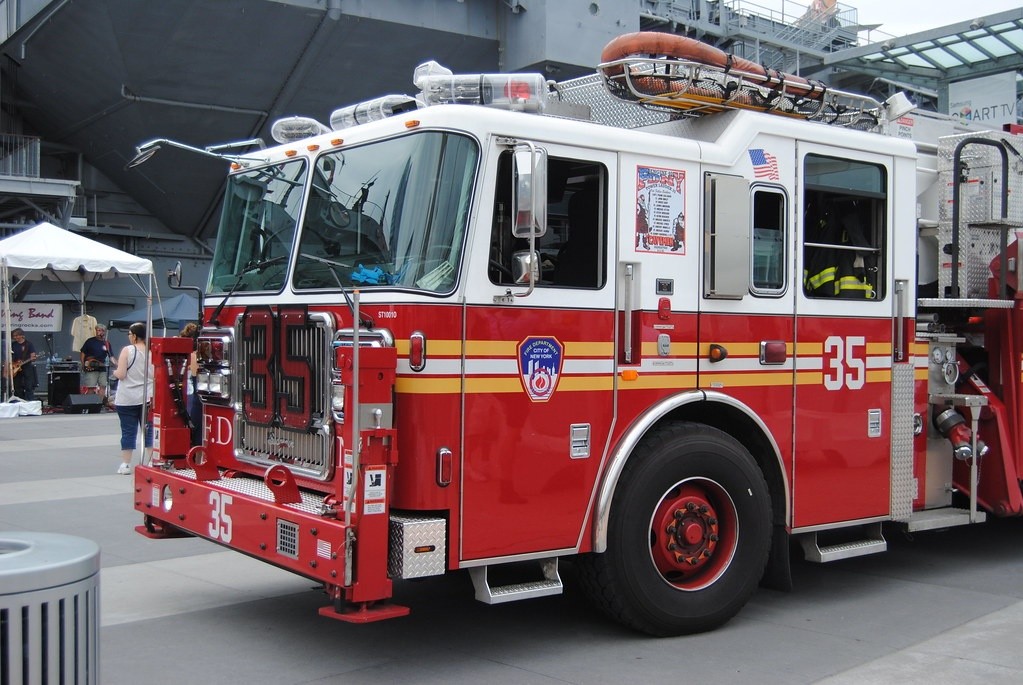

(0, 529), (106, 685)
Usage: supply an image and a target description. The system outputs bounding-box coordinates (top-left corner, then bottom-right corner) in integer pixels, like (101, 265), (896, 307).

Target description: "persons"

(11, 328), (39, 401)
(173, 323), (204, 447)
(112, 322), (156, 475)
(80, 324), (117, 401)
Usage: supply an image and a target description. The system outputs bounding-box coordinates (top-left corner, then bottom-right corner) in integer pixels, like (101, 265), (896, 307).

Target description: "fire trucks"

(121, 28), (1023, 637)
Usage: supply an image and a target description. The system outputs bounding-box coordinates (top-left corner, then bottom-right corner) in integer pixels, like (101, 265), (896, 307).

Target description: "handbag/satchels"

(109, 375), (118, 390)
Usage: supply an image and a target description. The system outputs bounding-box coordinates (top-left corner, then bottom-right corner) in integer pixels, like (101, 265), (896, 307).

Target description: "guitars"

(84, 358), (110, 372)
(2, 350), (46, 379)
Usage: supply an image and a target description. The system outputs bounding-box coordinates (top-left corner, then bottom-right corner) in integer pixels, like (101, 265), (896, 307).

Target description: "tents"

(105, 295), (199, 341)
(0, 221), (156, 466)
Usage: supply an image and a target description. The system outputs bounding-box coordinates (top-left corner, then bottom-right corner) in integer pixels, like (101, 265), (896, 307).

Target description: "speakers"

(62, 394), (103, 415)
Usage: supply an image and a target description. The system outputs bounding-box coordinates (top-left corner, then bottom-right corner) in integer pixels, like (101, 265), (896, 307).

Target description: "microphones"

(44, 335), (50, 341)
(100, 333), (104, 337)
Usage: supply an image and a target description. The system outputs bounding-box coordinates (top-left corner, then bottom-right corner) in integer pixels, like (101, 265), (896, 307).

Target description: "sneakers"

(149, 461), (152, 466)
(117, 463), (131, 474)
